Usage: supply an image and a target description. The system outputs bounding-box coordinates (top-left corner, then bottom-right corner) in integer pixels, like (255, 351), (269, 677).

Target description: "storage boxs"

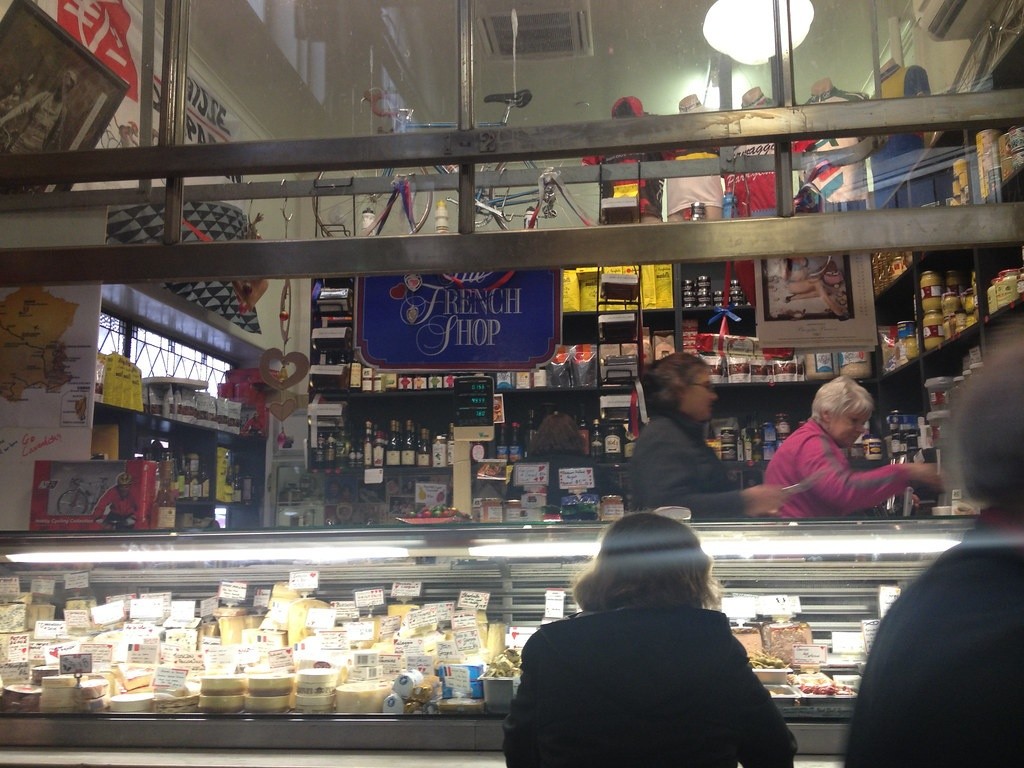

(30, 458), (157, 533)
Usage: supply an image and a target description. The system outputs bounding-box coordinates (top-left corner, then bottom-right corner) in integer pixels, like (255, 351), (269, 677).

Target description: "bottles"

(523, 205), (539, 230)
(433, 199), (450, 234)
(723, 185), (738, 219)
(89, 340), (877, 532)
(359, 207), (377, 237)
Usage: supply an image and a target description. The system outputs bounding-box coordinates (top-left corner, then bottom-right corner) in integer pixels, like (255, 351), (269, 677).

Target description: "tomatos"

(406, 505), (456, 518)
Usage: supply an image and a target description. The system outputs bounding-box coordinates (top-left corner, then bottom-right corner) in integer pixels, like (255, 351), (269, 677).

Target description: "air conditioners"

(912, 0), (998, 40)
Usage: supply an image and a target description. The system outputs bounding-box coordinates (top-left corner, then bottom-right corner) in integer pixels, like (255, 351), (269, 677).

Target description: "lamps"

(702, 0), (814, 66)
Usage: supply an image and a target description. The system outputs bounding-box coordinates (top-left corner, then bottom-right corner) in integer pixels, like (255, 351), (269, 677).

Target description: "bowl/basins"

(752, 668), (794, 684)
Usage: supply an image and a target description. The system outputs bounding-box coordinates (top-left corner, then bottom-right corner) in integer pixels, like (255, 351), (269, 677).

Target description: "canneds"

(890, 266), (1024, 455)
(945, 125), (1024, 205)
(840, 420), (884, 460)
(600, 495), (625, 522)
(721, 412), (793, 461)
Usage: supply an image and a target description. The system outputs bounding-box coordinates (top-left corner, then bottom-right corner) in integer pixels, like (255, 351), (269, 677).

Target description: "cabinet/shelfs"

(307, 22), (1024, 528)
(92, 399), (257, 535)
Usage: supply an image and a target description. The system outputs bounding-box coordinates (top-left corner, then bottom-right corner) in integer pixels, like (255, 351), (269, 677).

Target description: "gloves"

(125, 515), (137, 526)
(95, 518), (109, 527)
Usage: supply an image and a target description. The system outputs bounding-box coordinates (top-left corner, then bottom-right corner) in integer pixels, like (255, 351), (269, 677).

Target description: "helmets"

(117, 473), (133, 485)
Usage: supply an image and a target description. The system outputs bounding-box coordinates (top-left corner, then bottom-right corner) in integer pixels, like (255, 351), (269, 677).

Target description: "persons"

(504, 414), (630, 520)
(581, 59), (951, 225)
(626, 353), (790, 522)
(781, 255), (850, 322)
(844, 324), (1024, 768)
(502, 513), (799, 768)
(762, 376), (950, 518)
(93, 473), (138, 531)
(0, 71), (83, 152)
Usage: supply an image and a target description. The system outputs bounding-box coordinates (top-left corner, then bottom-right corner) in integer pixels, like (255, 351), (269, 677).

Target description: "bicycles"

(57, 477), (111, 516)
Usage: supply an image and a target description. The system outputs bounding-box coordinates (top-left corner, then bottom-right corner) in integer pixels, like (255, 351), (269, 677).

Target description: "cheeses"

(732, 622), (813, 665)
(0, 582), (504, 716)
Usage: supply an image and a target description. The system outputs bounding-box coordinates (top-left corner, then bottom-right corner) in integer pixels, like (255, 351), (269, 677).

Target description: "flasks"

(691, 202), (707, 221)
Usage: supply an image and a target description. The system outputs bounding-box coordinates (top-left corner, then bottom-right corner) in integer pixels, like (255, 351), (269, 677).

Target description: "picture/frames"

(0, 0), (130, 196)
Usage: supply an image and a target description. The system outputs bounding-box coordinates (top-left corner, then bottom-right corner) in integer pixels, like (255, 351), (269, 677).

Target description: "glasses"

(691, 381), (716, 390)
